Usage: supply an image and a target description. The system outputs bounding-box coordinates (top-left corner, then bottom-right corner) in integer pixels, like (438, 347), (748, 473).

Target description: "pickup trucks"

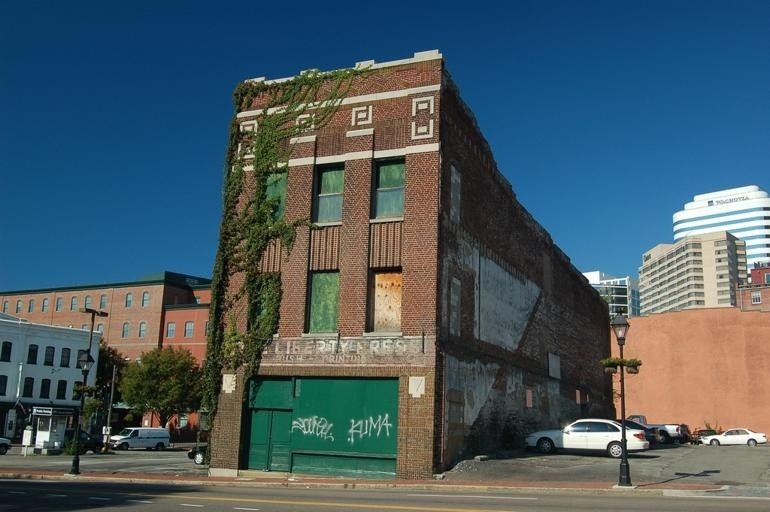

(626, 415), (682, 443)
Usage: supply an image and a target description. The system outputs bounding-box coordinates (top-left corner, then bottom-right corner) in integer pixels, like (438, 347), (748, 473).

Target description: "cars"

(613, 419), (659, 447)
(702, 428), (768, 448)
(524, 419), (650, 459)
(0, 438), (12, 456)
(188, 446), (207, 465)
(65, 429), (105, 454)
(697, 429), (717, 444)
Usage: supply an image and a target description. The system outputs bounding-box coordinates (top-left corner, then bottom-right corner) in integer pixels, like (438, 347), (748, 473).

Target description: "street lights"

(609, 313), (630, 488)
(69, 351), (95, 475)
(103, 354), (132, 443)
(67, 307), (109, 454)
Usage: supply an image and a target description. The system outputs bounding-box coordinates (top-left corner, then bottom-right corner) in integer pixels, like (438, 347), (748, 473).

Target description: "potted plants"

(600, 356), (620, 374)
(620, 358), (642, 374)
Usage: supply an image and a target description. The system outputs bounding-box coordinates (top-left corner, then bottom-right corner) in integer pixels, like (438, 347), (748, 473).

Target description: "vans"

(109, 428), (170, 451)
